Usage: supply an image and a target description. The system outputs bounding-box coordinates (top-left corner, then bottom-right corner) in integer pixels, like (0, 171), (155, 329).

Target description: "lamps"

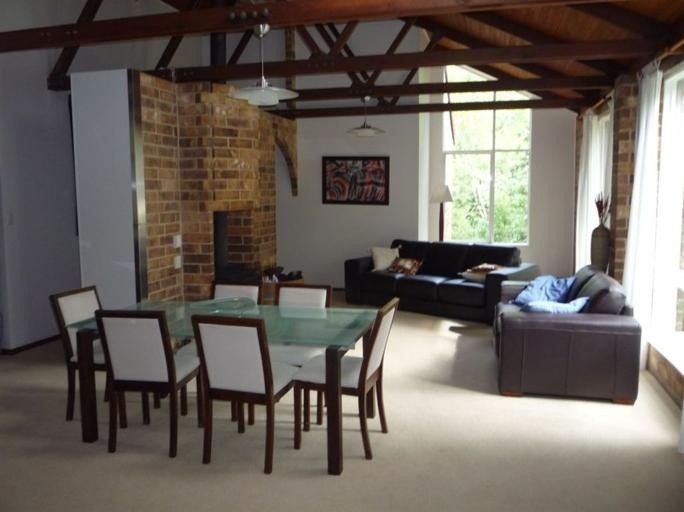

(345, 95), (386, 138)
(229, 24), (299, 105)
(429, 184), (454, 242)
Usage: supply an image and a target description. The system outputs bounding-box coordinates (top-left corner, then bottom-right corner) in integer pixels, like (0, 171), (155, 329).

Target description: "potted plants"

(591, 193), (612, 274)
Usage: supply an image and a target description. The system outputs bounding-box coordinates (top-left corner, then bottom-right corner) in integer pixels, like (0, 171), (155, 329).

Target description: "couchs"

(344, 239), (540, 327)
(492, 264), (641, 406)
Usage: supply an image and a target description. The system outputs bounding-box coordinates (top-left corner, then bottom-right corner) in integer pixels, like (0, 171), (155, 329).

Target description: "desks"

(75, 300), (380, 476)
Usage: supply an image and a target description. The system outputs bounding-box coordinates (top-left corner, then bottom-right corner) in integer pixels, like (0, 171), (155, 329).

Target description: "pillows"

(457, 264), (507, 284)
(364, 245), (402, 273)
(508, 274), (577, 307)
(519, 297), (590, 314)
(386, 256), (424, 276)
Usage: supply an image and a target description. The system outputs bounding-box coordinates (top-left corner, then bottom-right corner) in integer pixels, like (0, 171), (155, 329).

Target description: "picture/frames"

(321, 155), (390, 206)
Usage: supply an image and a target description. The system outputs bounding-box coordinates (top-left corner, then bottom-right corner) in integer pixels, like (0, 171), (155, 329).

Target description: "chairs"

(291, 297), (399, 461)
(180, 279), (264, 422)
(247, 282), (333, 433)
(191, 313), (302, 474)
(49, 284), (128, 429)
(95, 309), (204, 458)
